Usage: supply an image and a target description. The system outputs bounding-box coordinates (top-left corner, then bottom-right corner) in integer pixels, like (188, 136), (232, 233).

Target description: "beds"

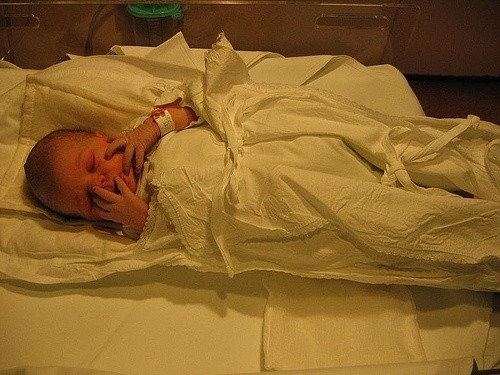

(1, 41), (499, 374)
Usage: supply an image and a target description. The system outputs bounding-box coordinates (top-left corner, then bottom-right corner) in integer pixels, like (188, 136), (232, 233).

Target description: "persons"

(24, 81), (499, 290)
